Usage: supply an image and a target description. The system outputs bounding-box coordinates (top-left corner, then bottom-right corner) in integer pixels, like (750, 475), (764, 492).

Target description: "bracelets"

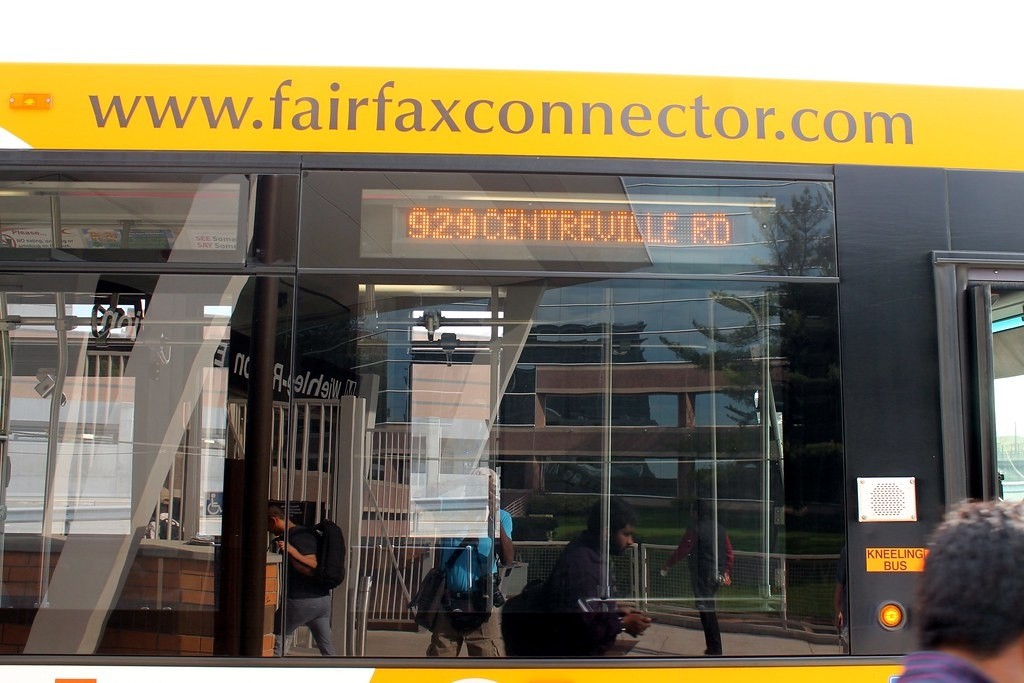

(618, 618), (627, 632)
(489, 515), (496, 525)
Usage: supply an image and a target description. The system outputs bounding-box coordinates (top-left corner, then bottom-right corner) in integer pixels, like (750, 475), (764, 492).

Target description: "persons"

(660, 500), (734, 656)
(834, 549), (848, 632)
(896, 500), (1023, 683)
(145, 487), (185, 541)
(499, 497), (654, 656)
(266, 505), (336, 656)
(410, 466), (514, 658)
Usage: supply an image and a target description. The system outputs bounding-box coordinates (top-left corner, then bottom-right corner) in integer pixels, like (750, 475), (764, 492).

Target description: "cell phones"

(641, 613), (657, 620)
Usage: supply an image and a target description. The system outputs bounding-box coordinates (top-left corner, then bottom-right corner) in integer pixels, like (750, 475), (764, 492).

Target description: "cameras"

(478, 572), (505, 608)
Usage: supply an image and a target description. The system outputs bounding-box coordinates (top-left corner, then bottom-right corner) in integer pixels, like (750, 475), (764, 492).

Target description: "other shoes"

(705, 649), (723, 655)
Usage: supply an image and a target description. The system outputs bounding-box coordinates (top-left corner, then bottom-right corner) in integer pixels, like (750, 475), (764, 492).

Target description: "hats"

(173, 488), (180, 498)
(161, 487), (169, 500)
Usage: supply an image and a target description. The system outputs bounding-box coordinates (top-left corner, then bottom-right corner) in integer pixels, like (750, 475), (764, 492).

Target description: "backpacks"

(272, 519), (346, 597)
(502, 579), (563, 657)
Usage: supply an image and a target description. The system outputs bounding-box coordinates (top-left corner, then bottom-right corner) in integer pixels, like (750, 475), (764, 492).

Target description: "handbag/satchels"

(407, 568), (445, 630)
(442, 588), (488, 633)
